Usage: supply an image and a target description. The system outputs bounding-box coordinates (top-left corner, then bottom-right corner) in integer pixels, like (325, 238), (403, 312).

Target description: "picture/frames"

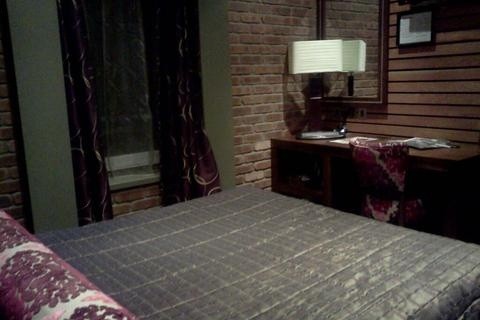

(396, 5), (436, 49)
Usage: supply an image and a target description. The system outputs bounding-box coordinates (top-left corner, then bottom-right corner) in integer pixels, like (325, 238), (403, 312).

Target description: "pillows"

(0, 208), (144, 319)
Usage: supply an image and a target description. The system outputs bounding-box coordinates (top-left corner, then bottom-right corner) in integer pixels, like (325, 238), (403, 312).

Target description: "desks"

(271, 133), (479, 223)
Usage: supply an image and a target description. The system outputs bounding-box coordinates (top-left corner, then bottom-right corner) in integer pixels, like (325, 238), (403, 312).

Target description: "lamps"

(287, 40), (367, 139)
(339, 41), (367, 97)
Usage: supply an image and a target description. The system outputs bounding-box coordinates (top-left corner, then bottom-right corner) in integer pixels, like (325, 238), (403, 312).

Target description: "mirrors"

(315, 0), (390, 114)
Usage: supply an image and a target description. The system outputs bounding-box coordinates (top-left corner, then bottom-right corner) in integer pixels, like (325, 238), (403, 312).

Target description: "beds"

(32, 184), (479, 319)
